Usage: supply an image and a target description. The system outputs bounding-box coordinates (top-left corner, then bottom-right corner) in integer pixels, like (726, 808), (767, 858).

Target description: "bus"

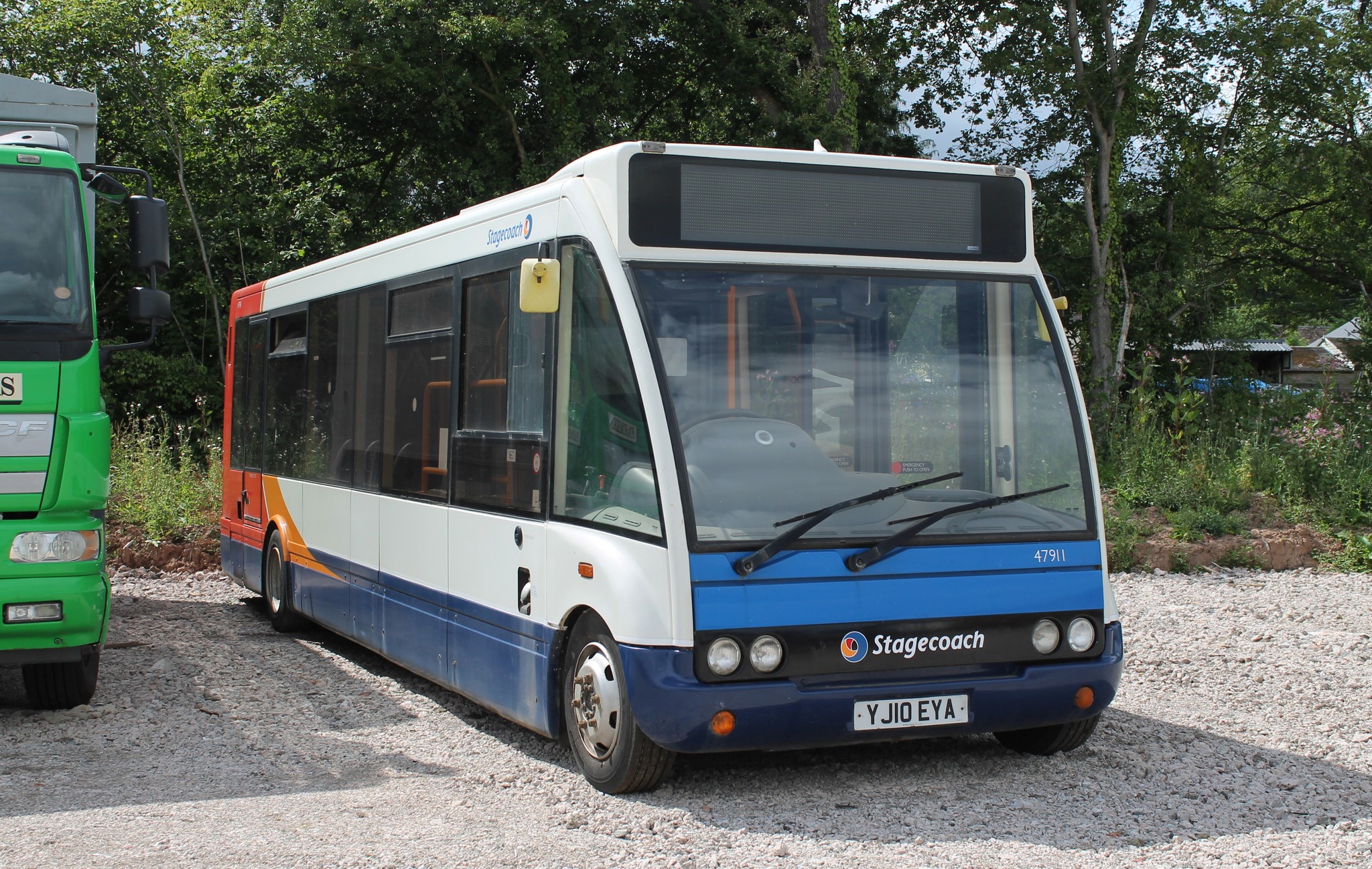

(220, 142), (1124, 787)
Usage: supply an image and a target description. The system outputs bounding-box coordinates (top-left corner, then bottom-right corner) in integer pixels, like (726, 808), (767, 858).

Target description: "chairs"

(315, 437), (418, 492)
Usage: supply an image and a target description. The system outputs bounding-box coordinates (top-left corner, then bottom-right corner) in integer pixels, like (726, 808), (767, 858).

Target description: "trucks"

(1, 64), (175, 705)
